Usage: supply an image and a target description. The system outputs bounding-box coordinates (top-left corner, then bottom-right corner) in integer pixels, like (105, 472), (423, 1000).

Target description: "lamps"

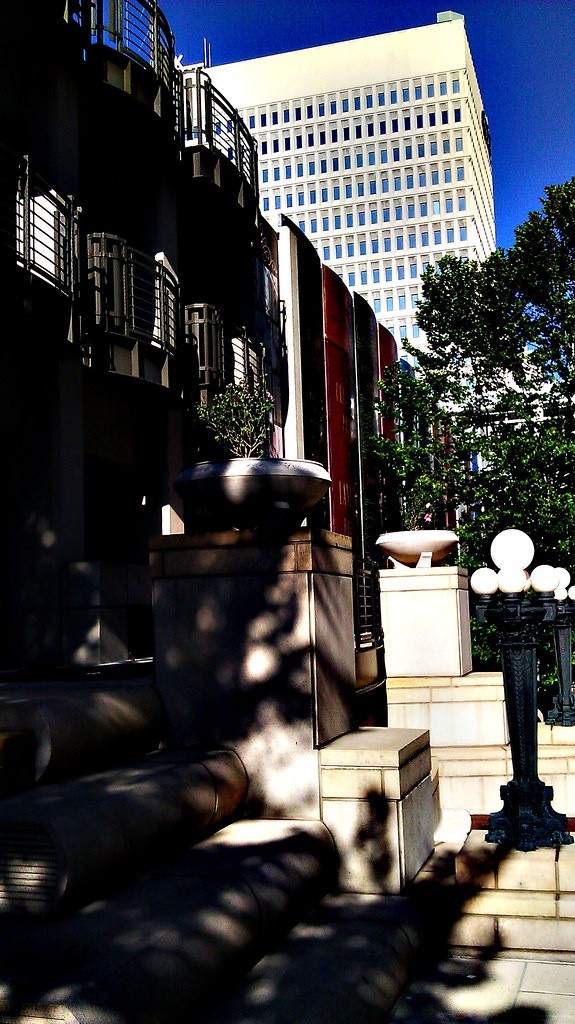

(469, 529), (575, 850)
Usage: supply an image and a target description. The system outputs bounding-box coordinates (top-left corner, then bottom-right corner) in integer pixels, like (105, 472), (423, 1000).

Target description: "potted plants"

(163, 375), (332, 532)
(375, 474), (460, 567)
